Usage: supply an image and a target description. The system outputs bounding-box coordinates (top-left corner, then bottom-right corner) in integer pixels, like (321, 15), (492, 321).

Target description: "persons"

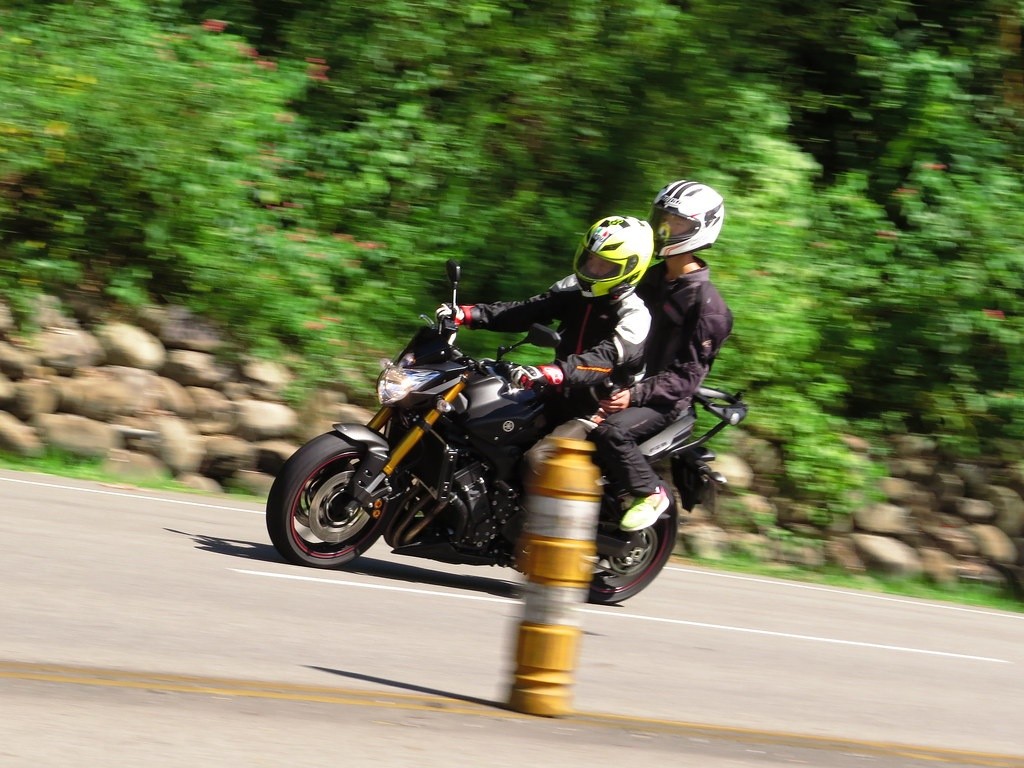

(434, 216), (654, 567)
(589, 179), (732, 531)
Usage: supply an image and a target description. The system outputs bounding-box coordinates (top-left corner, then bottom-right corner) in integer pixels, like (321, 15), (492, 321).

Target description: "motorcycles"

(265, 258), (749, 606)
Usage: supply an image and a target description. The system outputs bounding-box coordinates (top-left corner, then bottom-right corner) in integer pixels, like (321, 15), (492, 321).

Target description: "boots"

(449, 480), (523, 559)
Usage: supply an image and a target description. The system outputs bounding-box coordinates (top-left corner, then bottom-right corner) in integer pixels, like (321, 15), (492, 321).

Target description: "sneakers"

(619, 486), (670, 532)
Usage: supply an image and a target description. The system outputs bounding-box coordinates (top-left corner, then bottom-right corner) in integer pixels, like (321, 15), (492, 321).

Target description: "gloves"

(434, 301), (465, 329)
(510, 365), (548, 392)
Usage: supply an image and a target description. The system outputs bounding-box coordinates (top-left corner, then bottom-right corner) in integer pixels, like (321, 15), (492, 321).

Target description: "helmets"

(572, 215), (655, 305)
(648, 179), (726, 259)
